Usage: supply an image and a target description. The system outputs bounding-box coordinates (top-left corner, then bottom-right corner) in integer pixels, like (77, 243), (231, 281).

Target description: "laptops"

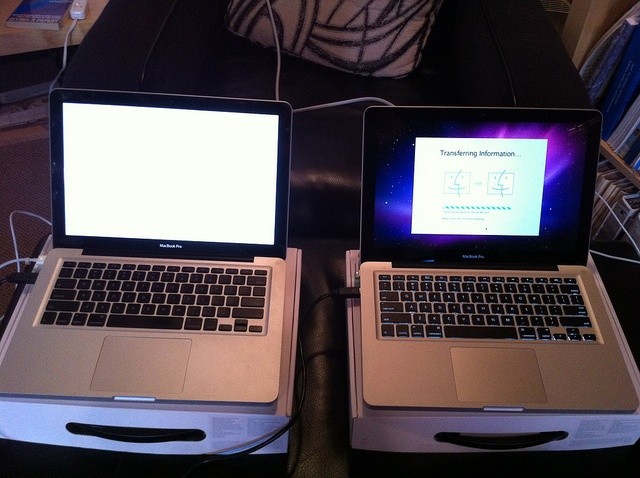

(0, 87), (294, 408)
(360, 105), (639, 414)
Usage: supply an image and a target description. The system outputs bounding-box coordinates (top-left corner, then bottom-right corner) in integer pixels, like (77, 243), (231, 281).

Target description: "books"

(590, 174), (640, 247)
(5, 0), (74, 31)
(574, 0), (640, 174)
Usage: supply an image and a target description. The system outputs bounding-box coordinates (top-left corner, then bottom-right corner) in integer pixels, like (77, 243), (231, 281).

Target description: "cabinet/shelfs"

(558, 0), (640, 244)
(1, 0), (109, 146)
